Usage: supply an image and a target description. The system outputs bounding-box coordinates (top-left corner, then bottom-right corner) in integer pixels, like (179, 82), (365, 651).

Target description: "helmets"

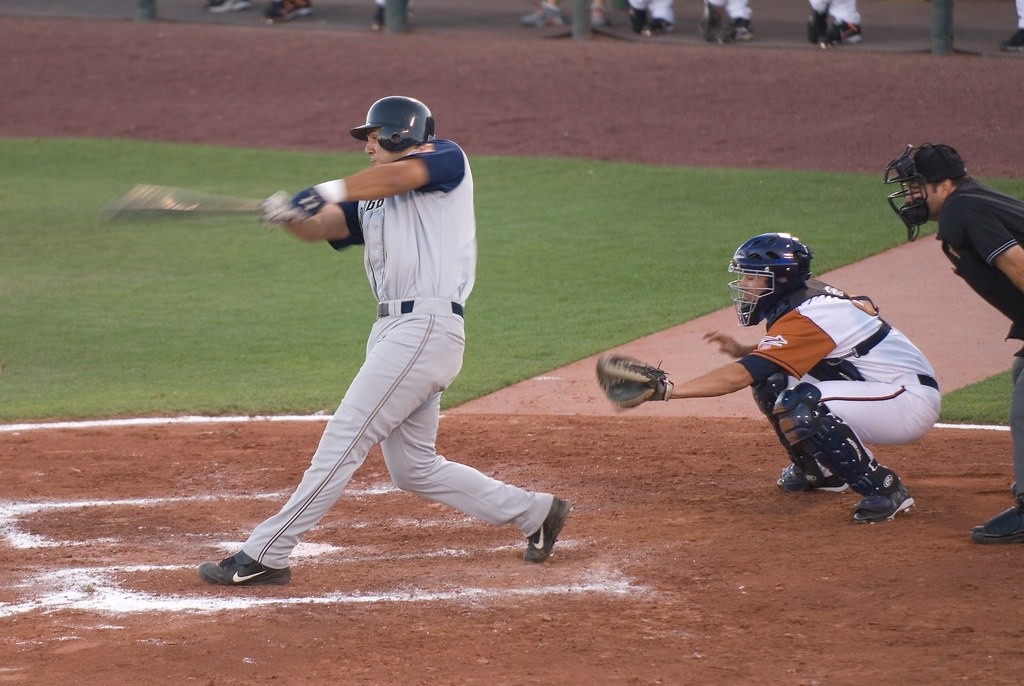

(732, 233), (814, 326)
(350, 96), (432, 151)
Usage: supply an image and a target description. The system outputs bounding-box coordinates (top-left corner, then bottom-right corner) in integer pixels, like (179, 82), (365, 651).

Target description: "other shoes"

(261, 0), (312, 23)
(818, 21), (862, 49)
(807, 11), (827, 44)
(969, 505), (1024, 544)
(590, 5), (609, 25)
(521, 3), (564, 25)
(735, 19), (755, 40)
(1003, 28), (1023, 53)
(628, 8), (648, 33)
(209, 0), (252, 14)
(639, 19), (675, 35)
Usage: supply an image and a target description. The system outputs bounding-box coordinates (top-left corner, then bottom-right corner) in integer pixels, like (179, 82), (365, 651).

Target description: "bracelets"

(314, 178), (346, 204)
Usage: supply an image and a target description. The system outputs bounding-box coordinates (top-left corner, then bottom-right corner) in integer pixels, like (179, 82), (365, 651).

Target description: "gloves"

(264, 185), (326, 223)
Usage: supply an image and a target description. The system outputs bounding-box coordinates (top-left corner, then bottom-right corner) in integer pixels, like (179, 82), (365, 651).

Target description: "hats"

(914, 144), (966, 181)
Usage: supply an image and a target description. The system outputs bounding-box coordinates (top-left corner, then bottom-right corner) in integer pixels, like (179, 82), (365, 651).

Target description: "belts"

(377, 301), (463, 318)
(917, 374), (939, 391)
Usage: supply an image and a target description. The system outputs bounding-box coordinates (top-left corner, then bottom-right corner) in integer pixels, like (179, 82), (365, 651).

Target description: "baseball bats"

(103, 183), (266, 218)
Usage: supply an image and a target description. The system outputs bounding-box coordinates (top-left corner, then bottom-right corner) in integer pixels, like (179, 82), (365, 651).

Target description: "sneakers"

(197, 549), (292, 586)
(810, 475), (849, 492)
(523, 496), (569, 562)
(853, 484), (915, 525)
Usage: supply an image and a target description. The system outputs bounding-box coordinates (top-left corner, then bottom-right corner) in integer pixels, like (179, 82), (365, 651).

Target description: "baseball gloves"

(591, 351), (674, 411)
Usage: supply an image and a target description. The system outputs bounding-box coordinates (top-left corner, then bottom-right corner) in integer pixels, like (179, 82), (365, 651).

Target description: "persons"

(207, 0), (314, 25)
(885, 142), (1024, 544)
(595, 233), (941, 524)
(521, 0), (610, 28)
(807, 0), (863, 48)
(699, 0), (753, 42)
(371, 0), (415, 31)
(999, 0), (1024, 51)
(199, 96), (570, 587)
(626, 0), (673, 38)
